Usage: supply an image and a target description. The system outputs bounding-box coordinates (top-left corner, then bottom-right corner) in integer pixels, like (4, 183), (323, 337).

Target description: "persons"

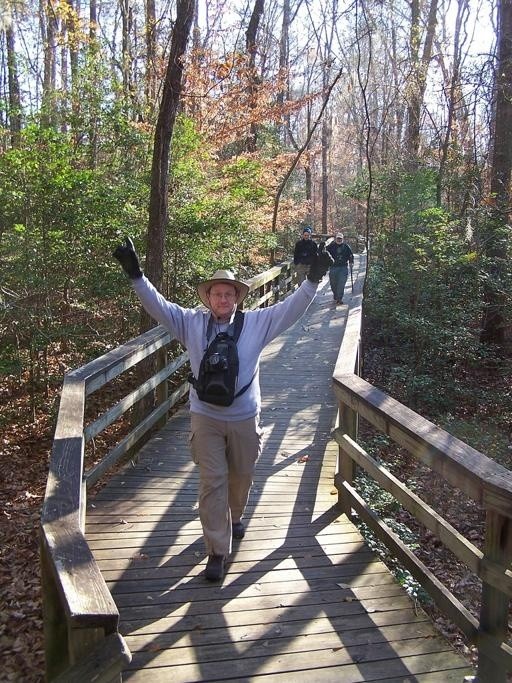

(294, 227), (317, 287)
(112, 236), (336, 582)
(326, 233), (354, 304)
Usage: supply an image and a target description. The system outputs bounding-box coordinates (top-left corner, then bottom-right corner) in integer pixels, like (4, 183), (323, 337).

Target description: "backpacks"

(197, 311), (245, 407)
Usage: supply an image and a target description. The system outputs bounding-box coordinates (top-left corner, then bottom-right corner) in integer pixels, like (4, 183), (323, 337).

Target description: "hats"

(336, 233), (343, 245)
(196, 270), (249, 310)
(303, 228), (311, 234)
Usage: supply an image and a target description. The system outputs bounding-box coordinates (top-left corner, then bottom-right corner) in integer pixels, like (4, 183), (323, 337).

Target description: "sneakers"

(232, 523), (245, 538)
(205, 555), (227, 580)
(333, 290), (343, 303)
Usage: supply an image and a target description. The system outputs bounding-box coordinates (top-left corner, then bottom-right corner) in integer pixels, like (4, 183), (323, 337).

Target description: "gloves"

(307, 241), (334, 283)
(112, 236), (142, 278)
